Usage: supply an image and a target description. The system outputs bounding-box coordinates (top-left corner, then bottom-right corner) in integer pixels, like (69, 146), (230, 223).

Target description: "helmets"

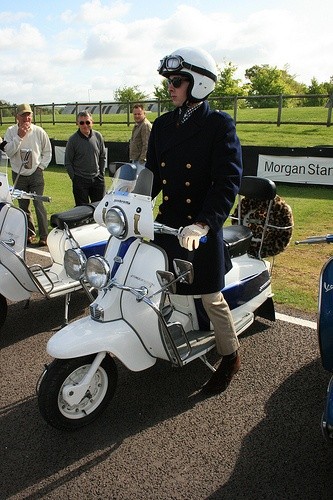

(160, 46), (217, 100)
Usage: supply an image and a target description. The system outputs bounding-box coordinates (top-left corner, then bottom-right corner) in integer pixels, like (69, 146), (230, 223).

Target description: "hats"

(17, 103), (32, 115)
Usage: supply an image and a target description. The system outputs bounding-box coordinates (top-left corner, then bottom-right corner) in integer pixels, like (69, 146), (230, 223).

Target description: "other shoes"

(205, 350), (242, 393)
(39, 235), (47, 246)
(28, 236), (35, 243)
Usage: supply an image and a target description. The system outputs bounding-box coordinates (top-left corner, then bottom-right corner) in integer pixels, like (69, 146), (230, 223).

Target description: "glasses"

(167, 76), (189, 88)
(80, 121), (91, 125)
(157, 54), (185, 74)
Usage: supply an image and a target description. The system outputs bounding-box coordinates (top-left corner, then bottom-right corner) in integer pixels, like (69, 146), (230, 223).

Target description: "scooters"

(295, 235), (333, 443)
(36, 162), (276, 433)
(0, 149), (112, 331)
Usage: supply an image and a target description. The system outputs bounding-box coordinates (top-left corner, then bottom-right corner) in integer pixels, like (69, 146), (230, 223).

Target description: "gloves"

(179, 222), (209, 252)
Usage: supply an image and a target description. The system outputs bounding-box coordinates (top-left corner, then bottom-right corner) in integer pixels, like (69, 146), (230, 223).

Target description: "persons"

(4, 104), (52, 245)
(129, 105), (152, 167)
(65, 111), (107, 206)
(133, 48), (243, 392)
